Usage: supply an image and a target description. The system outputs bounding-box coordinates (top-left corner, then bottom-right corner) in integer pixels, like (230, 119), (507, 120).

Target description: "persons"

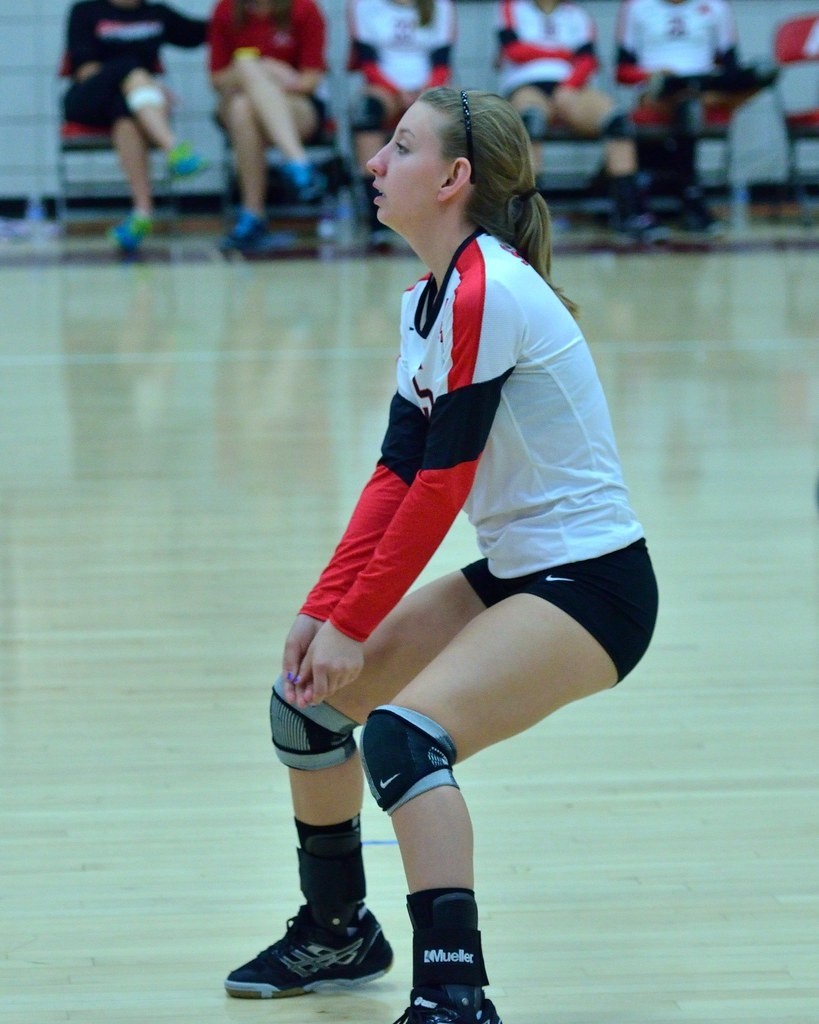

(225, 88), (659, 1024)
(57, 0), (775, 256)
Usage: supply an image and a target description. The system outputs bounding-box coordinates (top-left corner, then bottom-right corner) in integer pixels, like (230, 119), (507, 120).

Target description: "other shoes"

(620, 214), (671, 244)
(685, 200), (725, 236)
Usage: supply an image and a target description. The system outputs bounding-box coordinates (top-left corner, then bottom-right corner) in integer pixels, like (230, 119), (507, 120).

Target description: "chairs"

(52, 16), (819, 238)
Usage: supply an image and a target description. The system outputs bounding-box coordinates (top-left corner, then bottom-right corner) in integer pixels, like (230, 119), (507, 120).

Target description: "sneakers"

(166, 143), (211, 173)
(290, 161), (325, 199)
(224, 902), (393, 998)
(104, 214), (151, 249)
(229, 213), (269, 240)
(391, 988), (503, 1024)
